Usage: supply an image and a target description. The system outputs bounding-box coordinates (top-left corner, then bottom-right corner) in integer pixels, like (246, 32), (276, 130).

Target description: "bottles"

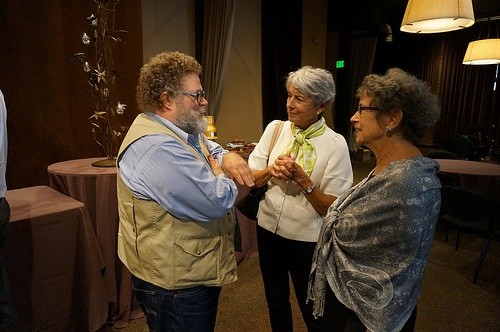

(476, 119), (496, 160)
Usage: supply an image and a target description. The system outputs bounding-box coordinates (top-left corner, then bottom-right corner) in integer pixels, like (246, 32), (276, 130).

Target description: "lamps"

(462, 0), (500, 65)
(399, 0), (475, 34)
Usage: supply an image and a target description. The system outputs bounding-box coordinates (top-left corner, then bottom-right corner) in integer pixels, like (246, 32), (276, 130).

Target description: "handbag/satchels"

(234, 119), (284, 222)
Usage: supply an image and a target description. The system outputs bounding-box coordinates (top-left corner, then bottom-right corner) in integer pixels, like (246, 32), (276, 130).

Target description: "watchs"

(300, 181), (315, 194)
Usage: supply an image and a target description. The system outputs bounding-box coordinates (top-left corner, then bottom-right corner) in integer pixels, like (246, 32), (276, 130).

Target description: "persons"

(0, 89), (10, 239)
(308, 69), (443, 332)
(116, 52), (255, 332)
(250, 65), (353, 332)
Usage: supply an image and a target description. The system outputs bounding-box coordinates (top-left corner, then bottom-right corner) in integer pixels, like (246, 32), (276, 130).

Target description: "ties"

(286, 115), (326, 178)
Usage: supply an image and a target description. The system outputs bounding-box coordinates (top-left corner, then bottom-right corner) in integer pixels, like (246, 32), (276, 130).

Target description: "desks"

(434, 158), (500, 195)
(0, 143), (257, 332)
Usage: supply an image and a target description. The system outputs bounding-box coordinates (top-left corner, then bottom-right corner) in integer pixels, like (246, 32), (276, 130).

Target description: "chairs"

(440, 184), (500, 284)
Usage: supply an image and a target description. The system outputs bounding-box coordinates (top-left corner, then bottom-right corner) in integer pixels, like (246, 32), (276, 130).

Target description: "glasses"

(357, 104), (394, 117)
(165, 90), (205, 103)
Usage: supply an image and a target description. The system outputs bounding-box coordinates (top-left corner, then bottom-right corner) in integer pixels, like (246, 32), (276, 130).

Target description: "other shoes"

(235, 250), (244, 263)
